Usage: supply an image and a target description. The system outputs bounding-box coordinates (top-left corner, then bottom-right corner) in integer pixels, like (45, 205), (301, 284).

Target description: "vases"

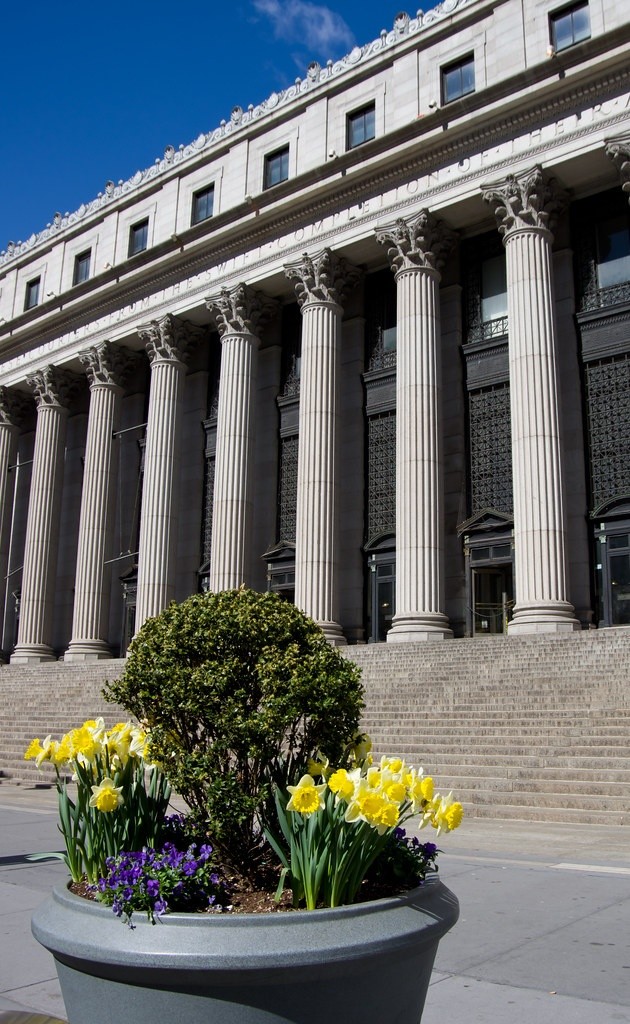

(27, 871), (458, 1024)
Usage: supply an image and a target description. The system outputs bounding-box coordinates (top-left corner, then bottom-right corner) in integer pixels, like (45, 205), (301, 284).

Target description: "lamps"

(245, 195), (253, 205)
(546, 44), (557, 58)
(328, 149), (338, 162)
(171, 232), (178, 243)
(429, 100), (440, 111)
(103, 263), (114, 270)
(0, 317), (7, 324)
(48, 291), (57, 300)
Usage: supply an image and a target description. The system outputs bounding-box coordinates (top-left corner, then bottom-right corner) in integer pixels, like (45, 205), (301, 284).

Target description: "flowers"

(23, 588), (459, 923)
